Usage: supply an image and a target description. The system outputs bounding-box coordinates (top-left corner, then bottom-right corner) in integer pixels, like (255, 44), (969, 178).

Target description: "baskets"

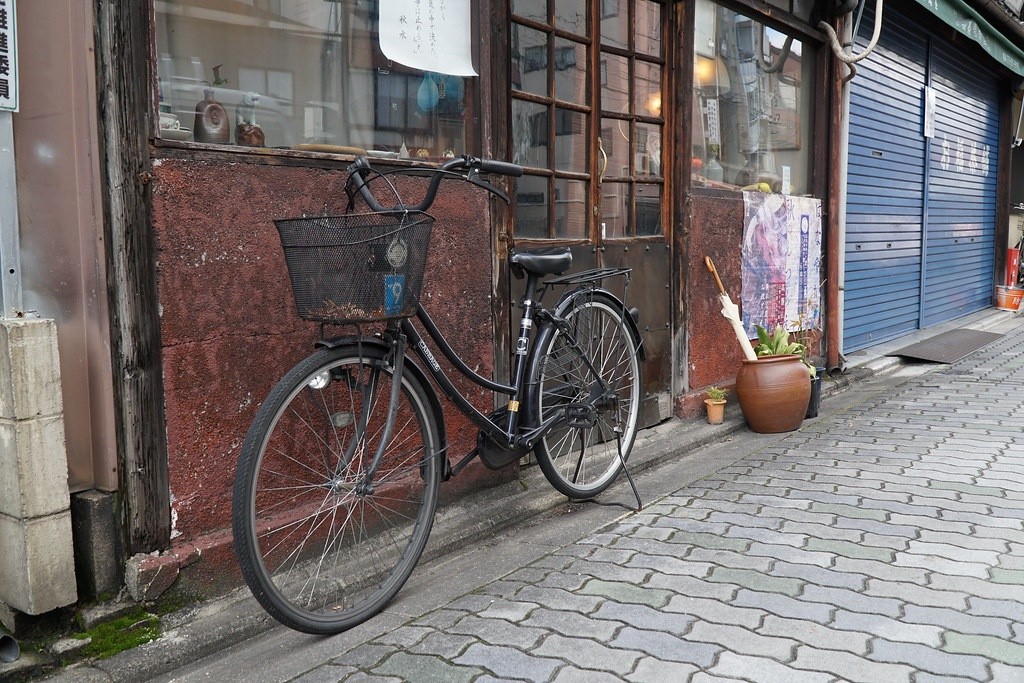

(272, 209), (436, 324)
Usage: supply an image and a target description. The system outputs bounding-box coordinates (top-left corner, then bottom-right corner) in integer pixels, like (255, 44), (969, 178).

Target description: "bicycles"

(230, 154), (649, 637)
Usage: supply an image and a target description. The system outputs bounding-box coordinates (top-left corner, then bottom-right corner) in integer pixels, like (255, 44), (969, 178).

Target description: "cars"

(158, 76), (287, 139)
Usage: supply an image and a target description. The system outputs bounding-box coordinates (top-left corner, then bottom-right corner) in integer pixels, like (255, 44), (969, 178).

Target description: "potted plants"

(703, 385), (731, 425)
(787, 278), (828, 419)
(702, 143), (724, 183)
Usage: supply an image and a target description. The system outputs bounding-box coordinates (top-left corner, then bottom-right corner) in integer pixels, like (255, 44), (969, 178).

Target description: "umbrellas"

(705, 256), (757, 360)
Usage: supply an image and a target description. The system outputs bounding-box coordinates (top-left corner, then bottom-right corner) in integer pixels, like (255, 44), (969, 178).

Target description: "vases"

(731, 350), (809, 435)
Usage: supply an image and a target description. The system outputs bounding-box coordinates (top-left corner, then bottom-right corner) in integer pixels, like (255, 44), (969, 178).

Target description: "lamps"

(643, 90), (661, 118)
(694, 51), (732, 97)
